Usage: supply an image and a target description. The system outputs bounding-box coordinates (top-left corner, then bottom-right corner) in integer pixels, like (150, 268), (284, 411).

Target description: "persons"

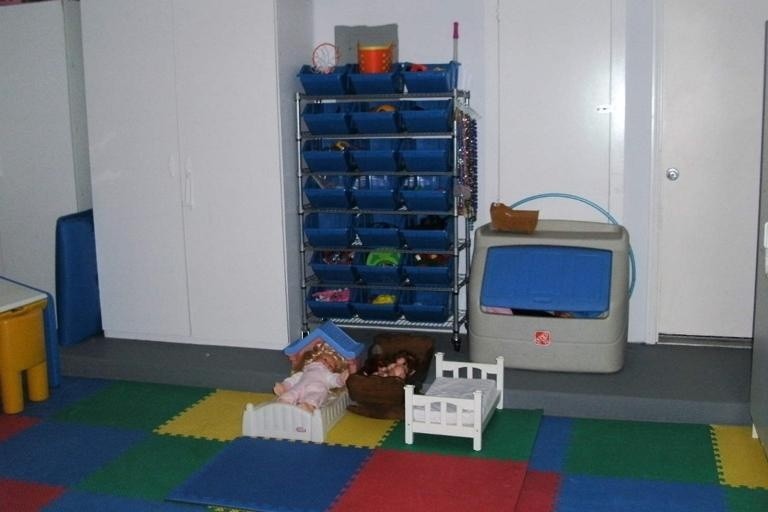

(273, 350), (352, 413)
(365, 351), (413, 381)
